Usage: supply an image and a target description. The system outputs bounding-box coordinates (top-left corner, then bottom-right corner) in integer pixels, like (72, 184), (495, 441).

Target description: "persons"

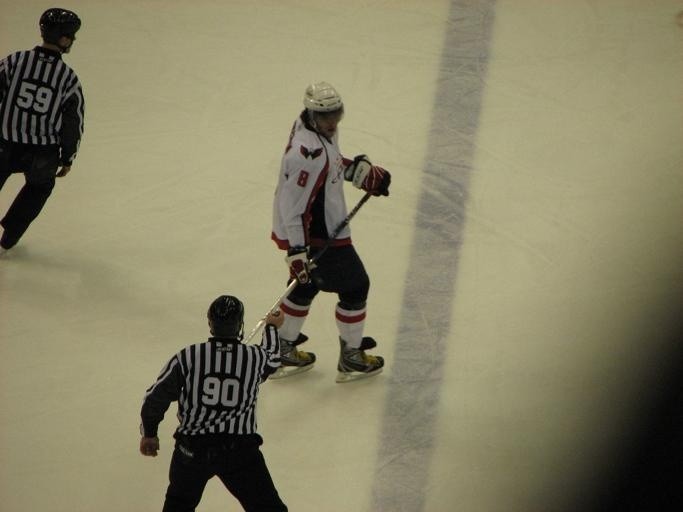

(268, 79), (391, 381)
(0, 7), (86, 252)
(138, 293), (287, 511)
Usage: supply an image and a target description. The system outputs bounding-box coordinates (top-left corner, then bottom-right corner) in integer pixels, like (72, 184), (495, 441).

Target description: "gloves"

(344, 153), (391, 197)
(283, 246), (322, 290)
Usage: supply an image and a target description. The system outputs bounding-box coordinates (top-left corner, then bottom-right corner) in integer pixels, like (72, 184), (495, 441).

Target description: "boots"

(280, 333), (316, 367)
(337, 335), (384, 373)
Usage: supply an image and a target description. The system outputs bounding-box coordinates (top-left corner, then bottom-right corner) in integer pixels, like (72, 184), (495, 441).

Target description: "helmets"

(207, 295), (245, 336)
(303, 80), (345, 134)
(38, 8), (82, 40)
